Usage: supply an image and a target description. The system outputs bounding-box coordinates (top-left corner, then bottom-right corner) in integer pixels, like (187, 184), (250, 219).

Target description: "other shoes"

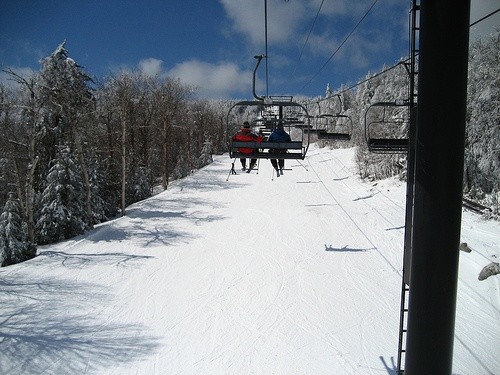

(280, 166), (283, 170)
(250, 164), (254, 168)
(275, 166), (279, 171)
(242, 163), (245, 167)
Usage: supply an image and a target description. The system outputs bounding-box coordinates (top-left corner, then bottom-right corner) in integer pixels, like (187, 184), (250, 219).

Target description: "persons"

(230, 121), (263, 169)
(267, 122), (291, 171)
(257, 130), (267, 141)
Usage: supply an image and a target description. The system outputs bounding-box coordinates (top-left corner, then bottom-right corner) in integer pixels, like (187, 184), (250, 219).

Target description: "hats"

(243, 122), (250, 128)
